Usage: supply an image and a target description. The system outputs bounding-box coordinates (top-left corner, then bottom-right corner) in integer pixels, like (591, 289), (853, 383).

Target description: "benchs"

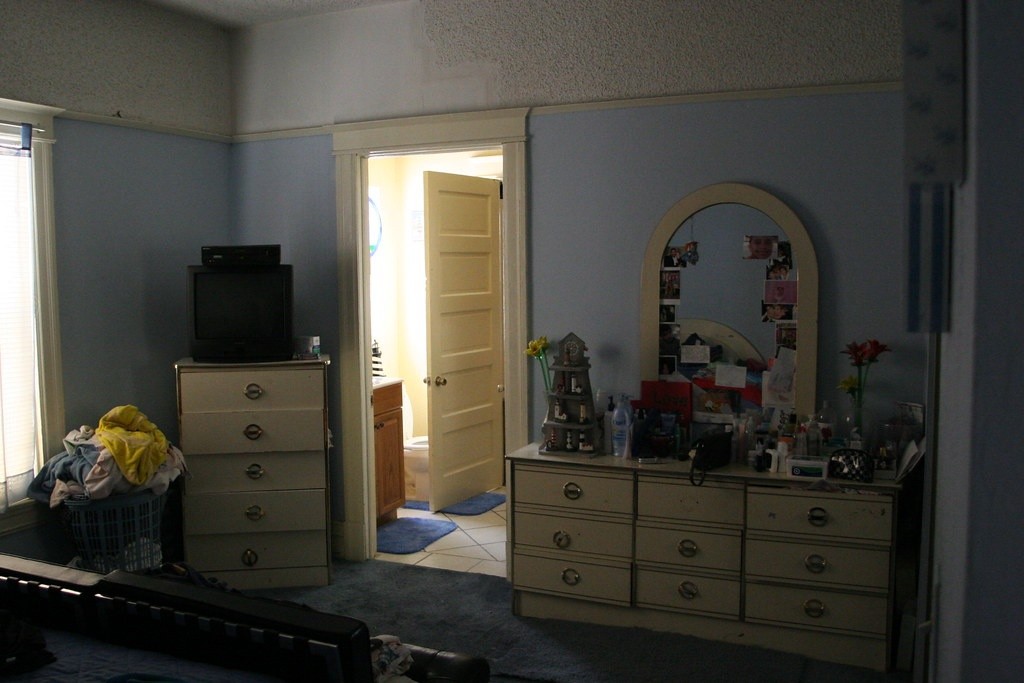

(1, 550), (492, 683)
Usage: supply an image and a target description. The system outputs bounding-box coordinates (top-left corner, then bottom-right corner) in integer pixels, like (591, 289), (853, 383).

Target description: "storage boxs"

(295, 335), (320, 354)
(785, 454), (829, 481)
(873, 460), (897, 480)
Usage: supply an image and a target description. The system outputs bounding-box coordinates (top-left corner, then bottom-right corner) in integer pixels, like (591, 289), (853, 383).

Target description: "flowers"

(524, 335), (554, 391)
(835, 339), (892, 438)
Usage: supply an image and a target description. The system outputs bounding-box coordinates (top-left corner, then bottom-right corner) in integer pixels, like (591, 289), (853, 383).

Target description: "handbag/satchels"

(689, 432), (733, 487)
(827, 449), (875, 484)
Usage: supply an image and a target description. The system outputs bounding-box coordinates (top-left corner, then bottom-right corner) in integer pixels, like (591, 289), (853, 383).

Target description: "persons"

(772, 284), (785, 304)
(663, 305), (674, 321)
(768, 264), (797, 281)
(664, 248), (683, 267)
(660, 325), (680, 358)
(664, 272), (674, 296)
(747, 236), (773, 258)
(762, 305), (791, 323)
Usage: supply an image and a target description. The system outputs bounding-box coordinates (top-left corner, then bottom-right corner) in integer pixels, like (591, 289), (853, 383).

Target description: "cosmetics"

(604, 394), (836, 480)
(548, 375), (588, 451)
(827, 415), (887, 482)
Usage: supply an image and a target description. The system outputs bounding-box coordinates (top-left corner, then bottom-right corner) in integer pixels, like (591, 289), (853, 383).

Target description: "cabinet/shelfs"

(174, 353), (333, 593)
(537, 332), (604, 459)
(504, 441), (924, 675)
(372, 376), (404, 529)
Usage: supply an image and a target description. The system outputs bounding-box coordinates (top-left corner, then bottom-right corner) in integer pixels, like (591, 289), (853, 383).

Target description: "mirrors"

(641, 183), (819, 427)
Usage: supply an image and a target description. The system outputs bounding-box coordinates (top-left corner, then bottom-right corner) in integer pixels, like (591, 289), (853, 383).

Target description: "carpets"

(376, 518), (457, 555)
(401, 487), (513, 521)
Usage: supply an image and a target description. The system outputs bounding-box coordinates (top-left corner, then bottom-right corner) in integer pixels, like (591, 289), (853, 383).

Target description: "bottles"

(752, 398), (836, 474)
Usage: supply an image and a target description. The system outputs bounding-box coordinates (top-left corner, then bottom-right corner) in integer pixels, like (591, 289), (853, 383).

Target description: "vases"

(846, 407), (866, 440)
